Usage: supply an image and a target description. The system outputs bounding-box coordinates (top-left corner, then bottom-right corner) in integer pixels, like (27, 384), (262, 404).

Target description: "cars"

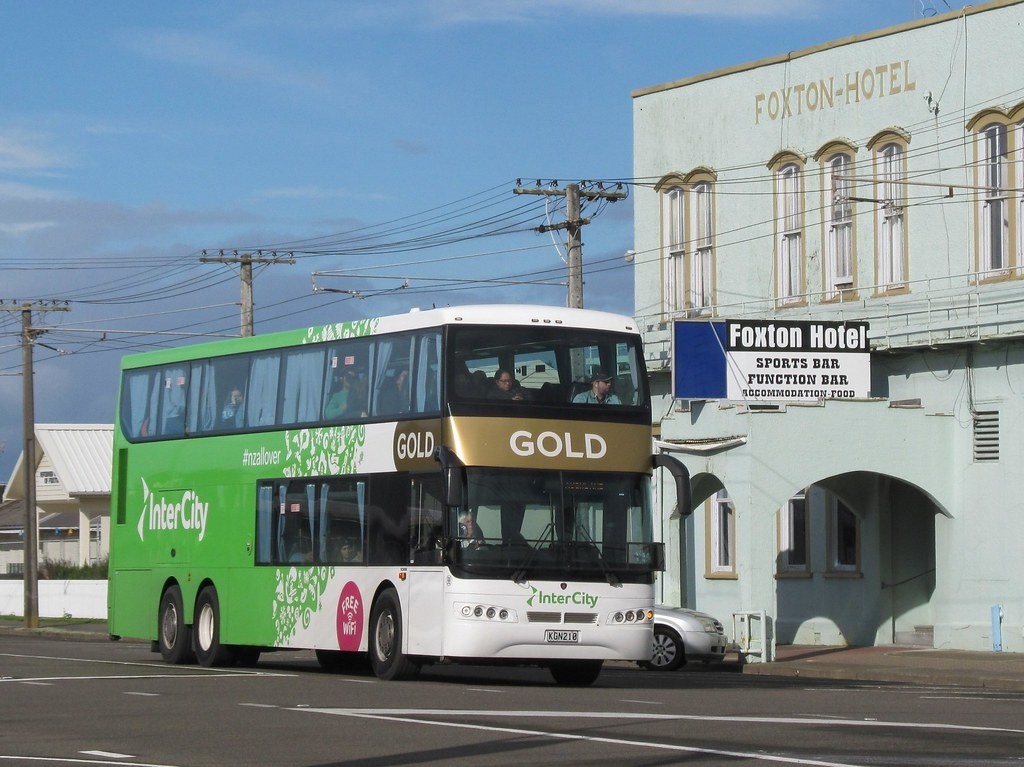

(640, 604), (726, 671)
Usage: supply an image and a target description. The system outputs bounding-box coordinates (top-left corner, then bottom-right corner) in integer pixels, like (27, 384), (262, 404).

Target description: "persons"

(333, 536), (356, 563)
(326, 367), (363, 419)
(458, 510), (486, 549)
(381, 366), (410, 415)
(485, 369), (533, 401)
(572, 369), (622, 405)
(221, 384), (244, 429)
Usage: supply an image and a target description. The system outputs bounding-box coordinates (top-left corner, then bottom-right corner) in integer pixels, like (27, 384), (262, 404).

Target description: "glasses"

(498, 380), (514, 384)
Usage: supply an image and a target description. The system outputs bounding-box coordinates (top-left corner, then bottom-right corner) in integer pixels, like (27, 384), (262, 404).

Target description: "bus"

(104, 303), (696, 687)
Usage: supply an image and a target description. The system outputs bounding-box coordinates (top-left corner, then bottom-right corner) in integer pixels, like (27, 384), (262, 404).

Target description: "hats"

(590, 374), (613, 383)
